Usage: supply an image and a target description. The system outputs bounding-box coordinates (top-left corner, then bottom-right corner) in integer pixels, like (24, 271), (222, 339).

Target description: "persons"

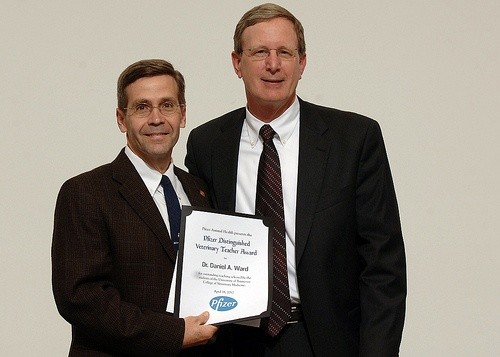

(51, 59), (218, 357)
(184, 3), (407, 357)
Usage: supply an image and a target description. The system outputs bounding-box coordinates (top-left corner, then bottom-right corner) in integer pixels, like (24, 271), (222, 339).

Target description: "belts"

(284, 310), (303, 325)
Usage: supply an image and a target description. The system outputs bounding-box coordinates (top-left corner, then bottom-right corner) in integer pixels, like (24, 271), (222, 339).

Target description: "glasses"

(124, 101), (182, 116)
(241, 46), (298, 60)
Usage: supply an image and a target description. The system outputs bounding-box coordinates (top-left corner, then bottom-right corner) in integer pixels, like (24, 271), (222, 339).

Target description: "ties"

(255, 125), (291, 338)
(159, 175), (181, 250)
